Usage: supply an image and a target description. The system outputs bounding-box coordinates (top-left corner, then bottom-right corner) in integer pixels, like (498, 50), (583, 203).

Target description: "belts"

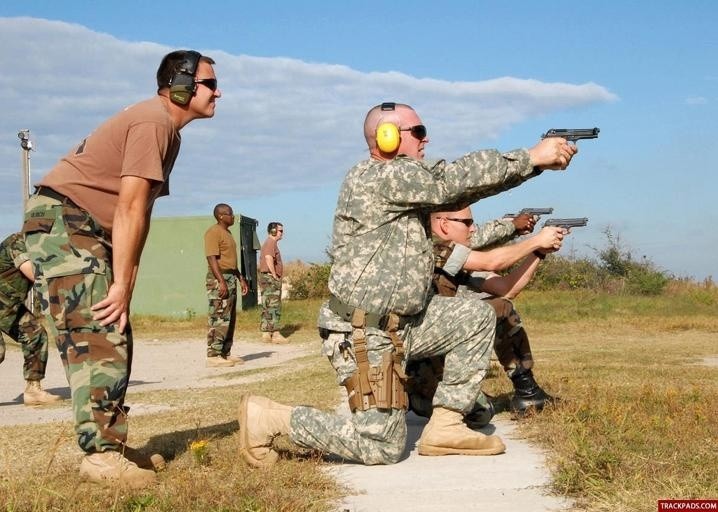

(218, 267), (245, 279)
(260, 270), (283, 280)
(327, 292), (427, 330)
(33, 185), (86, 213)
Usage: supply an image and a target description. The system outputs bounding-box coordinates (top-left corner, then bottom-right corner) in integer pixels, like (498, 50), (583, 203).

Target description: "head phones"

(271, 222), (276, 236)
(168, 50), (201, 107)
(374, 102), (401, 155)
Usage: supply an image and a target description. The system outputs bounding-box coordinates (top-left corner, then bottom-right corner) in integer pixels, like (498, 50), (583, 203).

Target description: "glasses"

(436, 214), (476, 229)
(195, 77), (220, 92)
(275, 228), (284, 233)
(399, 125), (429, 142)
(221, 212), (234, 217)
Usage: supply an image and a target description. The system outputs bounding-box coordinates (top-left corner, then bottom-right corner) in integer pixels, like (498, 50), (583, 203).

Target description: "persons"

(205, 203), (249, 367)
(22, 50), (220, 483)
(1, 231), (58, 405)
(463, 207), (552, 246)
(258, 223), (291, 345)
(238, 103), (579, 470)
(410, 205), (570, 428)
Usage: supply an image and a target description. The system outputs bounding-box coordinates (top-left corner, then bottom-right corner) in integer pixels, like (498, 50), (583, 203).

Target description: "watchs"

(534, 250), (547, 259)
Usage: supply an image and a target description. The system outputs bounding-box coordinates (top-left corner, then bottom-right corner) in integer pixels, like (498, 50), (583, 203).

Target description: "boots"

(113, 444), (170, 471)
(206, 356), (236, 370)
(226, 353), (246, 367)
(22, 380), (63, 406)
(272, 331), (294, 346)
(79, 453), (160, 491)
(418, 409), (506, 456)
(261, 331), (272, 343)
(237, 391), (293, 471)
(508, 369), (569, 420)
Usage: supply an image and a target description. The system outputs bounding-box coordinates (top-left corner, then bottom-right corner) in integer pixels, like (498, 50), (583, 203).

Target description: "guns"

(541, 217), (587, 232)
(542, 128), (600, 144)
(520, 207), (552, 221)
